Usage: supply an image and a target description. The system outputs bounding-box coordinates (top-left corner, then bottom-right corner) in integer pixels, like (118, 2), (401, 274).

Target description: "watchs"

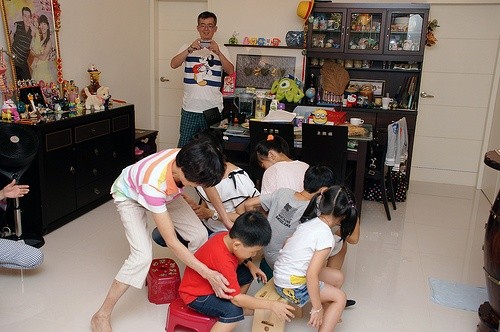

(212, 210), (218, 221)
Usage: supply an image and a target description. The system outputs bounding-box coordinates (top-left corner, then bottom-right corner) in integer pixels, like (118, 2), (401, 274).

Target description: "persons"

(10, 7), (53, 85)
(179, 132), (360, 332)
(170, 11), (234, 148)
(89, 132), (236, 332)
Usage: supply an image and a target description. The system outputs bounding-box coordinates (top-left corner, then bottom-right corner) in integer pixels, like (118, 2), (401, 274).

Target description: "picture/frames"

(0, 0), (64, 113)
(348, 79), (385, 97)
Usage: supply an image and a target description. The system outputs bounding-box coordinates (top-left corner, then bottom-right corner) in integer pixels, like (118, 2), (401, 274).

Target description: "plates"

(380, 106), (392, 110)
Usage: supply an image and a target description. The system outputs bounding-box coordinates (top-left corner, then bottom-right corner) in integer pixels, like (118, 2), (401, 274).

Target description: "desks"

(136, 129), (159, 163)
(209, 116), (374, 225)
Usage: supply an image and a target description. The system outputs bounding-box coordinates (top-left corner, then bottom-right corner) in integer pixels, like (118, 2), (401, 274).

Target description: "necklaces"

(300, 193), (311, 200)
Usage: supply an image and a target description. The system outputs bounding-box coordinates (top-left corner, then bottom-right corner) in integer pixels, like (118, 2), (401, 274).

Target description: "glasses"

(199, 24), (216, 28)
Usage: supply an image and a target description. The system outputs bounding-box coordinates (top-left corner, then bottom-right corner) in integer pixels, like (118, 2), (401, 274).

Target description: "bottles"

(343, 83), (373, 103)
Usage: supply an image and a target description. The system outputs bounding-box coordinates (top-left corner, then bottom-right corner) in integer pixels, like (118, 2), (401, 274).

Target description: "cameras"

(201, 39), (211, 47)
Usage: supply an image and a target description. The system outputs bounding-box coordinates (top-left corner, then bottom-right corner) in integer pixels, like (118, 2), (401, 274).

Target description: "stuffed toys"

(84, 87), (110, 111)
(271, 73), (305, 103)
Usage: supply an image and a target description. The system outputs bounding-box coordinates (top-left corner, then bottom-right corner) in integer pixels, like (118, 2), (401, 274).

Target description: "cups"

(278, 103), (286, 111)
(68, 93), (79, 104)
(309, 17), (421, 51)
(350, 117), (364, 126)
(382, 98), (393, 108)
(233, 92), (256, 118)
(374, 98), (382, 106)
(295, 115), (305, 126)
(309, 57), (374, 70)
(255, 97), (266, 119)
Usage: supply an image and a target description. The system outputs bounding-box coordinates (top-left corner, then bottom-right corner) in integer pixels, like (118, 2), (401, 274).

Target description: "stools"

(251, 276), (304, 332)
(145, 258), (181, 305)
(165, 296), (219, 332)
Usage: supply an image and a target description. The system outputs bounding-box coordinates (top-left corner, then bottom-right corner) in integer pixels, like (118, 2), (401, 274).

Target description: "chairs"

(301, 122), (354, 190)
(248, 121), (296, 193)
(199, 107), (256, 205)
(346, 116), (408, 221)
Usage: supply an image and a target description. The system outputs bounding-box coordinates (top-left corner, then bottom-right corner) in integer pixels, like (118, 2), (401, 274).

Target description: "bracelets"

(309, 304), (322, 314)
(241, 257), (252, 266)
(187, 47), (192, 53)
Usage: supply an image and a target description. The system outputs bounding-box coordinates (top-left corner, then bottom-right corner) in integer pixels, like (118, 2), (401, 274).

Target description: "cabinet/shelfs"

(0, 99), (135, 237)
(300, 2), (431, 202)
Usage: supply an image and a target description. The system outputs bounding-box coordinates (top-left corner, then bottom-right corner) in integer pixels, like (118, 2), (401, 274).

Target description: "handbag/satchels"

(364, 168), (409, 202)
